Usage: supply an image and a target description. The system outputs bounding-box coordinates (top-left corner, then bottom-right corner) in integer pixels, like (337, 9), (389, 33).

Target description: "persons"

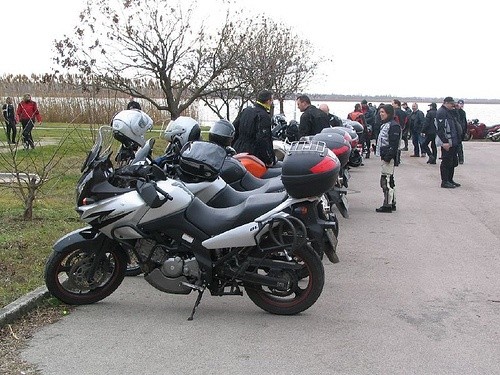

(347, 99), (468, 164)
(17, 92), (42, 150)
(435, 97), (462, 188)
(376, 104), (402, 212)
(232, 90), (276, 168)
(296, 94), (329, 137)
(318, 104), (343, 128)
(127, 96), (141, 110)
(2, 96), (19, 144)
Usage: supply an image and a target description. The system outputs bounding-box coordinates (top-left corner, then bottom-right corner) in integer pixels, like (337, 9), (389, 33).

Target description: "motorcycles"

(464, 119), (500, 142)
(44, 123), (341, 322)
(105, 111), (366, 293)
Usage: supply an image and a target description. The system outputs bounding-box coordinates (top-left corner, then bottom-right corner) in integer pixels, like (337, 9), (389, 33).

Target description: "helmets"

(208, 119), (235, 146)
(171, 116), (200, 148)
(112, 108), (153, 149)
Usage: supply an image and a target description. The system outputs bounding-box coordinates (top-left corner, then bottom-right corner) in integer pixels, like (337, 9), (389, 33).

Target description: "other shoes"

(376, 205), (396, 213)
(421, 152), (426, 157)
(427, 155), (436, 165)
(401, 147), (408, 150)
(410, 154), (419, 157)
(441, 178), (461, 188)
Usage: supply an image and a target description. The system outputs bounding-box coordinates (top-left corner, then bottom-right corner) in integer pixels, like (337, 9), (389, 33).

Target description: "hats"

(444, 97), (455, 103)
(402, 103), (408, 106)
(428, 103), (437, 106)
